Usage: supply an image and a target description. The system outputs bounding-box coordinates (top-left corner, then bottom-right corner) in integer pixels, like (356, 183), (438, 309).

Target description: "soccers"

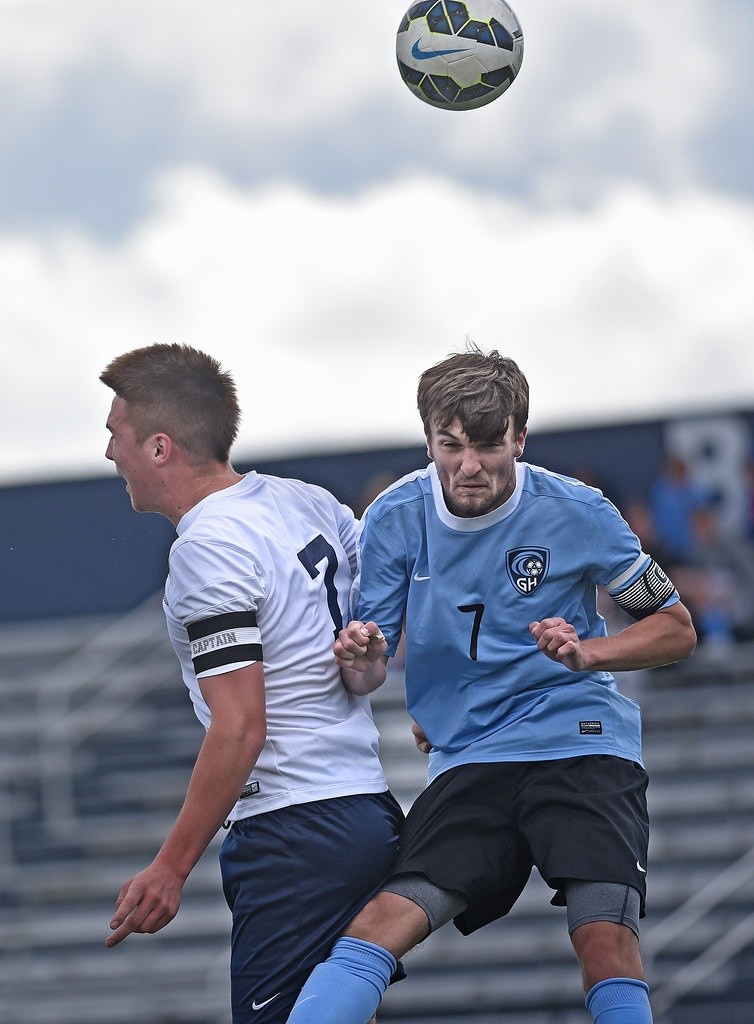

(395, 0), (525, 112)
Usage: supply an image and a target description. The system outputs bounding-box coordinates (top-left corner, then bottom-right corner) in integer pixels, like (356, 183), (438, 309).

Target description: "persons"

(285, 351), (697, 1024)
(560, 449), (754, 660)
(98, 344), (406, 1024)
